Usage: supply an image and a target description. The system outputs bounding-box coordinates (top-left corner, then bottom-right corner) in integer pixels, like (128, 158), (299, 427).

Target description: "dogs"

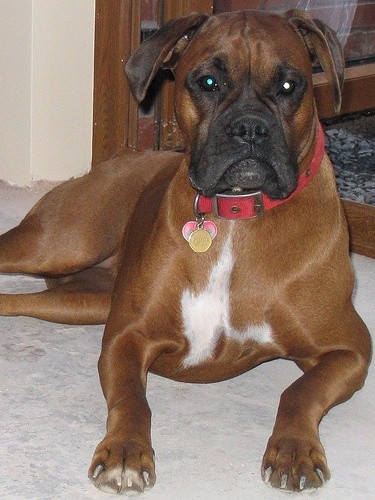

(0, 7), (373, 493)
